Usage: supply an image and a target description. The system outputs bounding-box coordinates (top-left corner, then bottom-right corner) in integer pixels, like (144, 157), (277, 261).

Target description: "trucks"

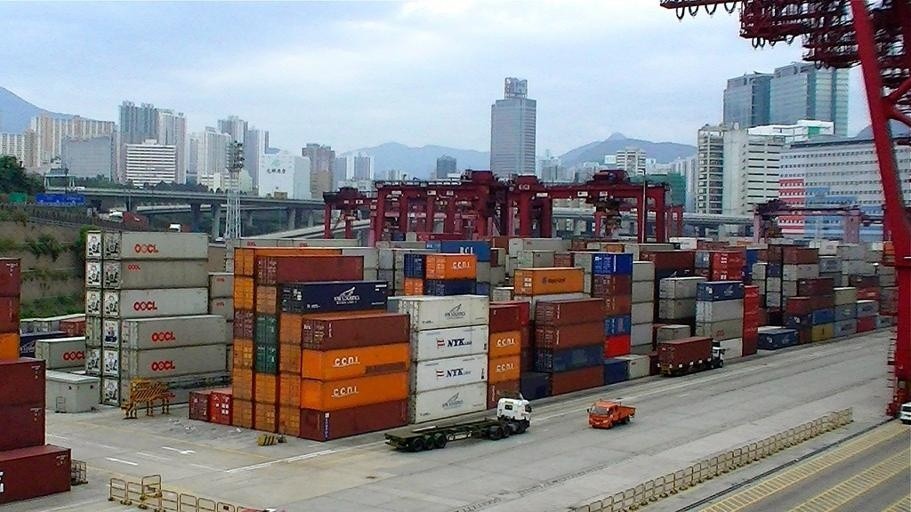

(380, 396), (533, 452)
(586, 397), (636, 429)
(654, 336), (727, 377)
